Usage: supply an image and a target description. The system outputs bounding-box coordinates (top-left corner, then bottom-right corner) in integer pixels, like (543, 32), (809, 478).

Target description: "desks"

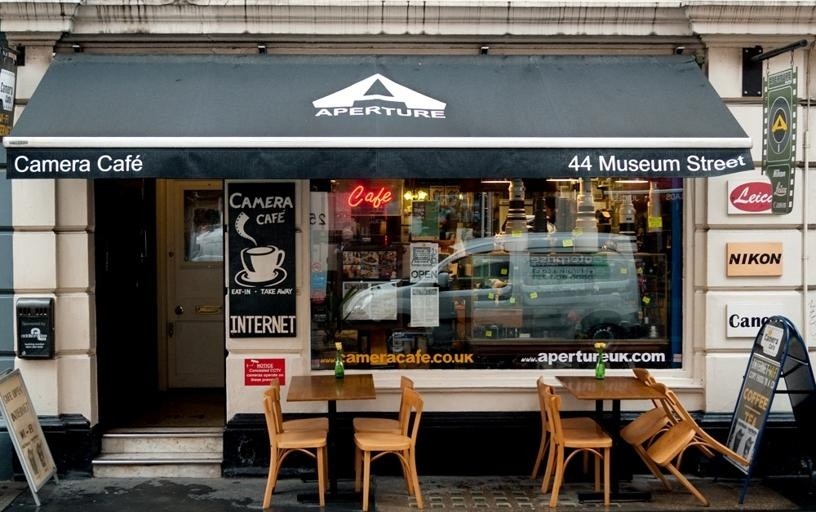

(284, 375), (377, 510)
(558, 375), (666, 506)
(454, 304), (525, 340)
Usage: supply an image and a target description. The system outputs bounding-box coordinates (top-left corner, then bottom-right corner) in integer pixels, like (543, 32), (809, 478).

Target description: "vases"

(335, 352), (345, 378)
(595, 353), (605, 380)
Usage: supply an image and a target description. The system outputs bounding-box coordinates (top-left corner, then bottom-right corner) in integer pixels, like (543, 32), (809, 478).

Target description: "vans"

(343, 233), (643, 354)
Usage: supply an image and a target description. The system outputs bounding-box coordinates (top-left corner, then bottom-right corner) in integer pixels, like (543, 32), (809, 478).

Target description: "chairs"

(622, 367), (716, 493)
(540, 391), (613, 507)
(351, 375), (415, 495)
(644, 380), (750, 507)
(353, 389), (428, 511)
(530, 377), (599, 495)
(259, 385), (330, 508)
(269, 376), (330, 493)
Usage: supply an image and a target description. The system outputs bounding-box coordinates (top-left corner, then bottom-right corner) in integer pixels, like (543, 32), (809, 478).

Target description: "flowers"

(593, 342), (607, 356)
(334, 341), (343, 351)
